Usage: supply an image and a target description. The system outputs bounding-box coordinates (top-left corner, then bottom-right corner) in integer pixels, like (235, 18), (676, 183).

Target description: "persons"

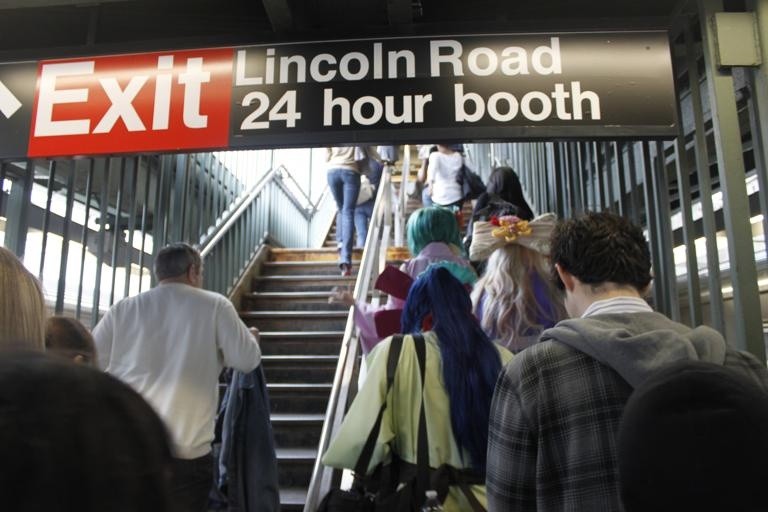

(1, 247), (49, 352)
(1, 350), (181, 511)
(45, 312), (94, 367)
(93, 238), (262, 512)
(326, 142), (766, 512)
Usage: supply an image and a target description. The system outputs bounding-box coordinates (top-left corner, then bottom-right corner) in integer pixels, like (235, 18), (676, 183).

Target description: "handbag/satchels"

(315, 482), (426, 510)
(406, 180), (424, 200)
(357, 174), (379, 207)
(459, 167), (486, 200)
